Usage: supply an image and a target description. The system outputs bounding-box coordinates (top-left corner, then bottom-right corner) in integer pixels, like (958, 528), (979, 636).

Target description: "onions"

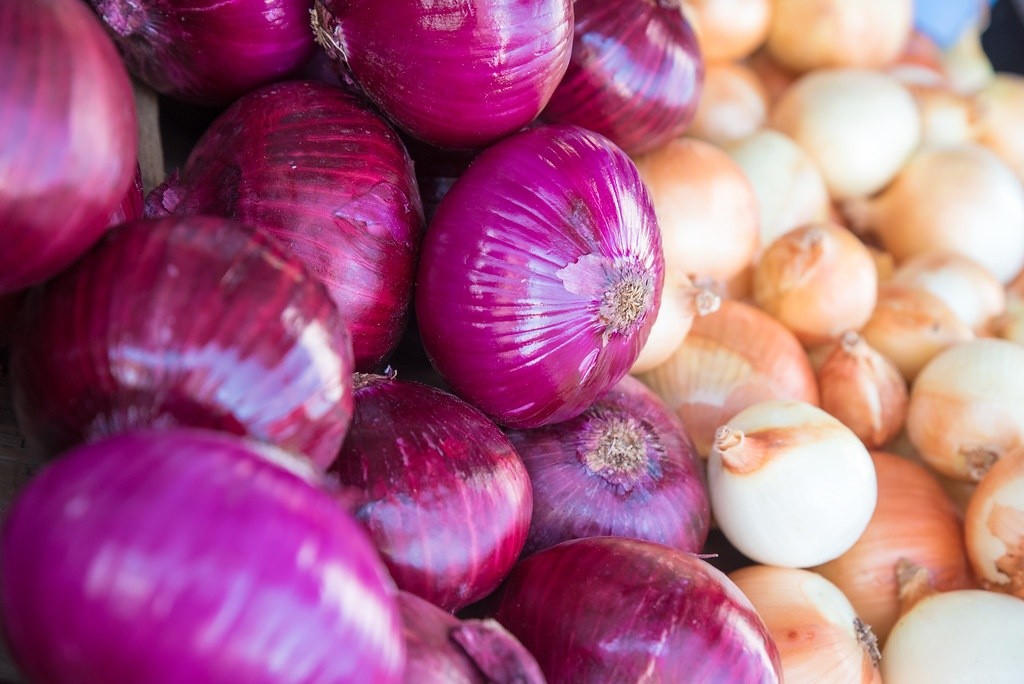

(0, 0), (1024, 684)
